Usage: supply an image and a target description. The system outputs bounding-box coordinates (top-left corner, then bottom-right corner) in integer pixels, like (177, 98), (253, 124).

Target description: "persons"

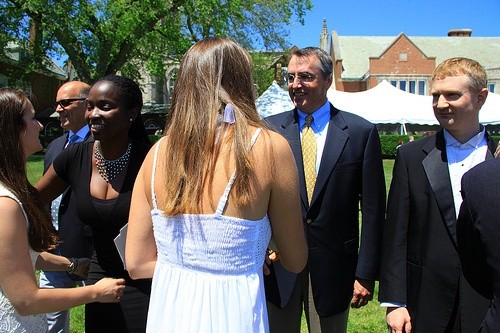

(1, 91), (126, 333)
(44, 82), (96, 172)
(261, 47), (387, 333)
(459, 140), (499, 333)
(33, 77), (156, 333)
(378, 58), (499, 333)
(125, 37), (308, 333)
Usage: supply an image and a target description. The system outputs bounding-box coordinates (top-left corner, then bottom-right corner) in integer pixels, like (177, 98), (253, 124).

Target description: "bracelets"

(68, 257), (79, 275)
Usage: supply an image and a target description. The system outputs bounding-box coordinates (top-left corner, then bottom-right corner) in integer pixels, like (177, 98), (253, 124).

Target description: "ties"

(51, 135), (79, 231)
(302, 116), (317, 206)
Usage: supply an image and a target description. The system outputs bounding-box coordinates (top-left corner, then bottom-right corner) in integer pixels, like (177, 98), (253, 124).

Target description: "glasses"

(54, 98), (86, 108)
(286, 73), (326, 83)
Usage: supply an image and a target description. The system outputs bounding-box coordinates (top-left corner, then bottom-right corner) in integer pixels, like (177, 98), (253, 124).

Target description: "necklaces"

(95, 142), (133, 184)
